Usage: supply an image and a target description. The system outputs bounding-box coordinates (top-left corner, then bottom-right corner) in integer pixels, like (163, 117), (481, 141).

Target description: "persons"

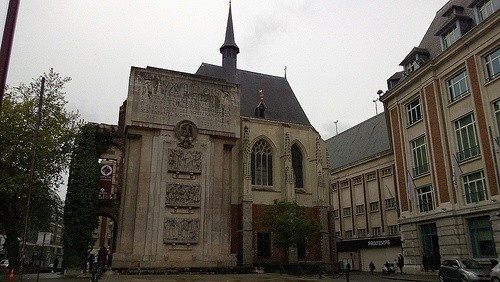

(394, 259), (397, 274)
(422, 252), (428, 272)
(369, 261), (375, 277)
(398, 253), (405, 274)
(429, 251), (437, 272)
(489, 257), (498, 269)
(53, 257), (59, 274)
(385, 262), (390, 275)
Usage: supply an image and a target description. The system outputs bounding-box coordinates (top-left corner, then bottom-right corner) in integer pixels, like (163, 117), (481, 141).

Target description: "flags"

(408, 174), (416, 200)
(453, 155), (461, 185)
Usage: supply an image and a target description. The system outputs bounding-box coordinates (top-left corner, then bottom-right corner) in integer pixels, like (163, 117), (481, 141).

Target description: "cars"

(438, 257), (490, 282)
(382, 263), (396, 275)
(489, 261), (500, 282)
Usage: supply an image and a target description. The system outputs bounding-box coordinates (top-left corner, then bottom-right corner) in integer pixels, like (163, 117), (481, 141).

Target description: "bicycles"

(91, 253), (104, 282)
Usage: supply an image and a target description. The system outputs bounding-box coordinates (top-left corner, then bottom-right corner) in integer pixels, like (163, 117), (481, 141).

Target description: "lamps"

(377, 90), (383, 95)
